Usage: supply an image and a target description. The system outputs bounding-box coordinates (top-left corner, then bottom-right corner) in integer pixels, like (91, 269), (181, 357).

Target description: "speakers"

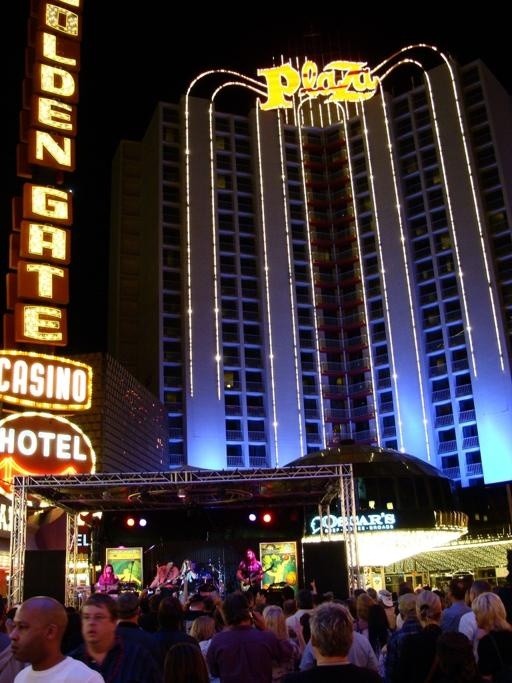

(23, 549), (66, 605)
(302, 540), (349, 599)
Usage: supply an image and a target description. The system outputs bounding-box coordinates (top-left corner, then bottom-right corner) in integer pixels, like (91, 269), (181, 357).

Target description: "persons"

(96, 559), (222, 605)
(236, 548), (264, 598)
(1, 579), (511, 681)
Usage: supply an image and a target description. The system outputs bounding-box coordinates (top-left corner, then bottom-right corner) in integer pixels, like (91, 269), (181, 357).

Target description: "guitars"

(241, 563), (274, 592)
(99, 576), (126, 594)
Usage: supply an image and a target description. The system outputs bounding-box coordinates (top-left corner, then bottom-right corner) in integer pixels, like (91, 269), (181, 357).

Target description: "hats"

(377, 591), (394, 607)
(113, 592), (141, 617)
